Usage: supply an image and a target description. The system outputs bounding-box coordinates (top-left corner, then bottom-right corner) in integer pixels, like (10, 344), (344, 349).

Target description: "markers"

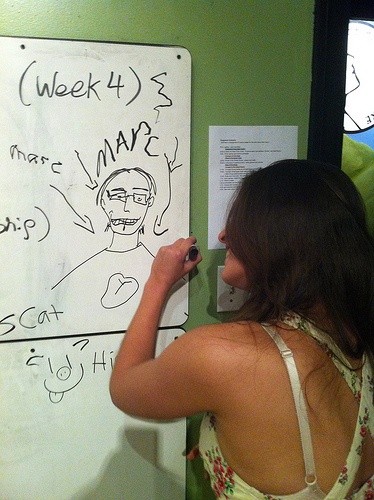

(186, 246), (197, 260)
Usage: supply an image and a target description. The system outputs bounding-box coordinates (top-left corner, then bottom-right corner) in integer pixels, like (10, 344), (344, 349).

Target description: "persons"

(108, 158), (374, 500)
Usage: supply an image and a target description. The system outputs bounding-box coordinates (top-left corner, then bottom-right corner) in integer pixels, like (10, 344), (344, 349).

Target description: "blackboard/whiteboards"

(0, 35), (190, 500)
